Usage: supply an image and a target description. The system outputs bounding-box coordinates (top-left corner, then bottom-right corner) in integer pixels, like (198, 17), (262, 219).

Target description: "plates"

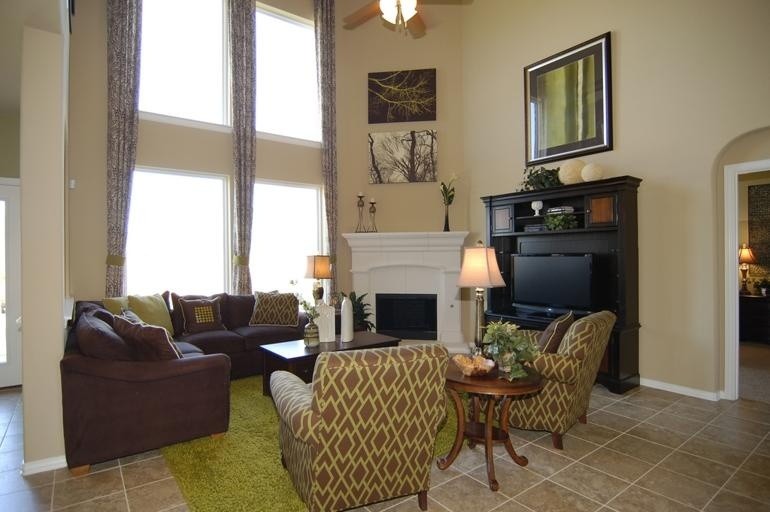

(453, 353), (496, 376)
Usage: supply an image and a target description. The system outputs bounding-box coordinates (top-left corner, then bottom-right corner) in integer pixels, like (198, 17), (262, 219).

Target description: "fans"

(342, 1), (474, 38)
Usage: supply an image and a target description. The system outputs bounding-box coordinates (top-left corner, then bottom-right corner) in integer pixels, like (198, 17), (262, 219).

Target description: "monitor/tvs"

(510, 252), (596, 320)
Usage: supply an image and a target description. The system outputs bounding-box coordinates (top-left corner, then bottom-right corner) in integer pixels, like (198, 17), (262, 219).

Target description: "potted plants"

(481, 321), (538, 382)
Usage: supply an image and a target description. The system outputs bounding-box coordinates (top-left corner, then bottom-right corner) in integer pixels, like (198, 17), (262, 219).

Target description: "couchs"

(472, 310), (618, 451)
(269, 345), (449, 512)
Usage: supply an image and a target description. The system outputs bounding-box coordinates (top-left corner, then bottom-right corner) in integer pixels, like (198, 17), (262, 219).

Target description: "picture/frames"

(523, 30), (612, 165)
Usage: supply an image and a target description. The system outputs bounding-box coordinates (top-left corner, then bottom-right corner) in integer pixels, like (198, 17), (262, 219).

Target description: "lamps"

(303, 255), (334, 300)
(458, 239), (507, 354)
(739, 244), (758, 295)
(379, 1), (417, 25)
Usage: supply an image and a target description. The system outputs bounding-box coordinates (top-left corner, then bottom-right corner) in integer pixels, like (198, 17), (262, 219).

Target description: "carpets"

(161, 374), (500, 512)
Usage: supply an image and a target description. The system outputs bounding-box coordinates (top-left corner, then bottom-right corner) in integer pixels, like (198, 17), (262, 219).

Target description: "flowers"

(439, 172), (458, 201)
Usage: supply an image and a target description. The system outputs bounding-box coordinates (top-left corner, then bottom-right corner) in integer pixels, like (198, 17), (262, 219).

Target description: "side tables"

(437, 353), (545, 491)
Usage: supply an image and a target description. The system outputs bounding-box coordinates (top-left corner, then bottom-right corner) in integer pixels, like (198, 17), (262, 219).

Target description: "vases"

(443, 202), (450, 231)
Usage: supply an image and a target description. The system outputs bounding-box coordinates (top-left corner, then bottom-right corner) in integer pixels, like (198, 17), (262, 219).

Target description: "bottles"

(340, 296), (354, 343)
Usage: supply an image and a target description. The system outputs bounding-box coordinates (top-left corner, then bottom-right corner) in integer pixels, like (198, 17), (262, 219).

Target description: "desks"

(259, 331), (401, 396)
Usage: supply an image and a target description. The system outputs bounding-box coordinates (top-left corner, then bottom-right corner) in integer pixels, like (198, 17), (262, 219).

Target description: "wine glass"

(531, 201), (543, 217)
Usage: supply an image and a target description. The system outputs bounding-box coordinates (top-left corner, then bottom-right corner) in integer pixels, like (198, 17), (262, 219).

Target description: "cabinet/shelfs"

(480, 176), (643, 395)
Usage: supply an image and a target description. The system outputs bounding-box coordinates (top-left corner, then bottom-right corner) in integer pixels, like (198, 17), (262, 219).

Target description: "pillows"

(74, 292), (184, 360)
(226, 290), (279, 328)
(537, 311), (573, 352)
(169, 290), (230, 334)
(249, 292), (301, 327)
